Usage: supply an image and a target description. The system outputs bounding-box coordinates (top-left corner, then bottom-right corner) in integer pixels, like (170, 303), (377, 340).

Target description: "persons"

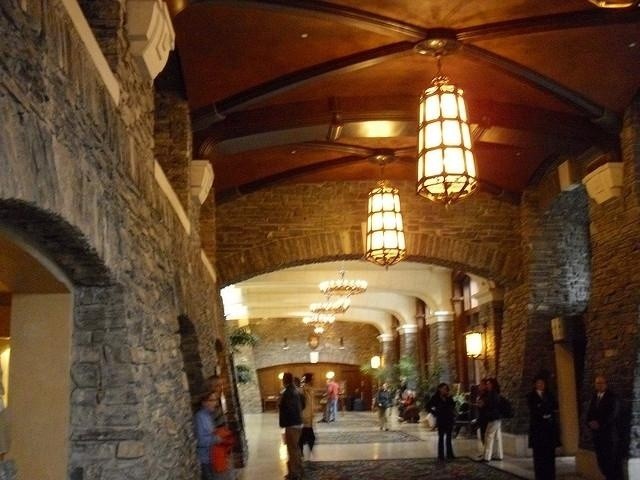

(476, 378), (488, 458)
(278, 371), (306, 480)
(303, 372), (315, 457)
(589, 374), (621, 479)
(527, 375), (564, 480)
(280, 376), (305, 396)
(318, 379), (421, 432)
(192, 390), (226, 479)
(423, 383), (459, 461)
(197, 374), (237, 479)
(477, 379), (512, 462)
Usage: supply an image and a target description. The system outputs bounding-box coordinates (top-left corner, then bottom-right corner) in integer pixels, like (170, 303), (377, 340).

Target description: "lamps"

(302, 270), (368, 335)
(463, 330), (487, 369)
(414, 38), (479, 209)
(364, 154), (407, 271)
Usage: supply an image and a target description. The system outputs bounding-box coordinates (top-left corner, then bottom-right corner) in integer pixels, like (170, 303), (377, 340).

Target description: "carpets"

(300, 456), (525, 480)
(312, 414), (425, 446)
